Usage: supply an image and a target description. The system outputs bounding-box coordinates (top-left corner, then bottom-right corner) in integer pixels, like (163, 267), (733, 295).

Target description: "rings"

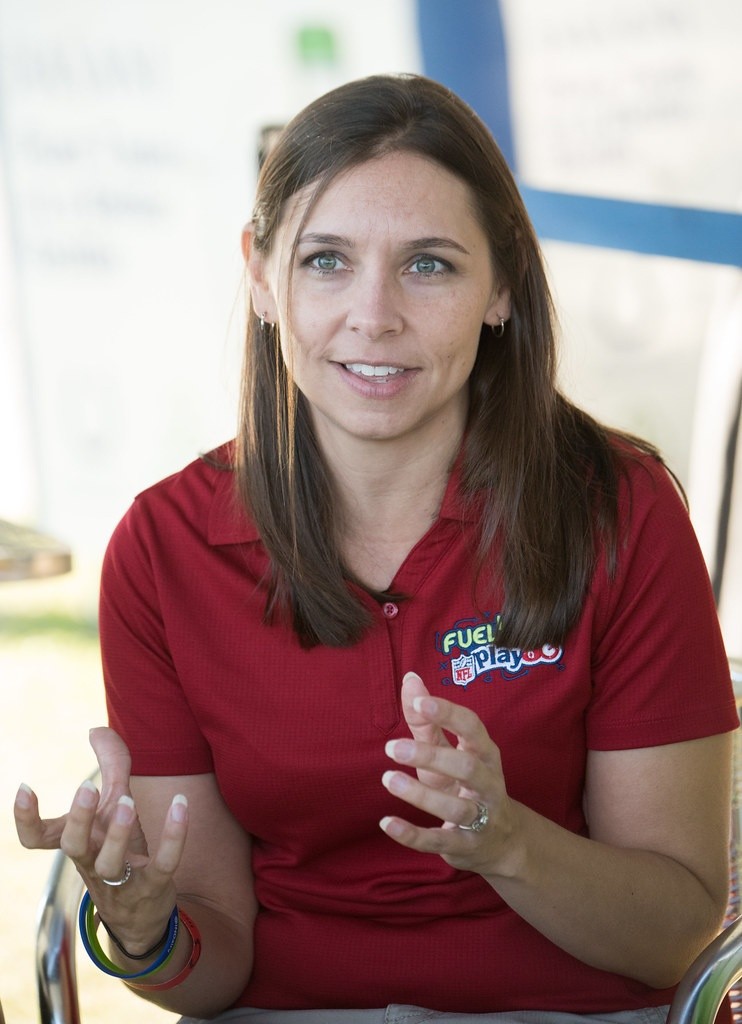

(101, 859), (132, 886)
(459, 800), (488, 831)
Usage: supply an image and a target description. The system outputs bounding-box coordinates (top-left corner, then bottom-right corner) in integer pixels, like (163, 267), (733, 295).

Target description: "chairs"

(37, 766), (742, 1024)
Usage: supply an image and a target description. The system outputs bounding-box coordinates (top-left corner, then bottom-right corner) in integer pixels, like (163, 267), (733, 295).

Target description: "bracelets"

(78, 888), (201, 992)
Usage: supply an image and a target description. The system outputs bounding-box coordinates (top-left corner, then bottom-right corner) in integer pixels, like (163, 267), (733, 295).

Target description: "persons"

(13, 72), (742, 1024)
